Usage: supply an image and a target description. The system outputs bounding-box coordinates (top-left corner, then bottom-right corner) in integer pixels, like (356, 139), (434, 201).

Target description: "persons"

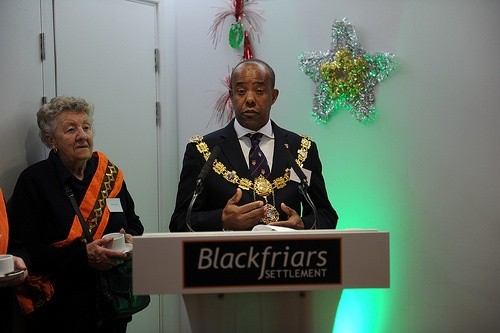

(168, 59), (341, 232)
(6, 97), (144, 333)
(0, 187), (27, 285)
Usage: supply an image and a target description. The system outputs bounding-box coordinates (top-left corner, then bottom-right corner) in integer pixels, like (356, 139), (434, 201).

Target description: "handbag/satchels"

(99, 259), (150, 321)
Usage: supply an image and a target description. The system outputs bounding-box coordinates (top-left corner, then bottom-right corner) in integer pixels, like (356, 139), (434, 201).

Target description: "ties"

(246, 132), (270, 181)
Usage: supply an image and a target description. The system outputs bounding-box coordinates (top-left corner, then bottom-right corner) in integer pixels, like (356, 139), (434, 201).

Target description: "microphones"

(186, 146), (221, 232)
(281, 145), (318, 230)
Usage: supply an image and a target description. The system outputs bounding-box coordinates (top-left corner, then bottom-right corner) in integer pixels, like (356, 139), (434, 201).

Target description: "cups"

(102, 233), (125, 253)
(0, 255), (14, 275)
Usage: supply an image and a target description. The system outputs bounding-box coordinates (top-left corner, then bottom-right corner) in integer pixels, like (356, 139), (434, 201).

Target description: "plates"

(102, 243), (133, 253)
(0, 270), (23, 278)
